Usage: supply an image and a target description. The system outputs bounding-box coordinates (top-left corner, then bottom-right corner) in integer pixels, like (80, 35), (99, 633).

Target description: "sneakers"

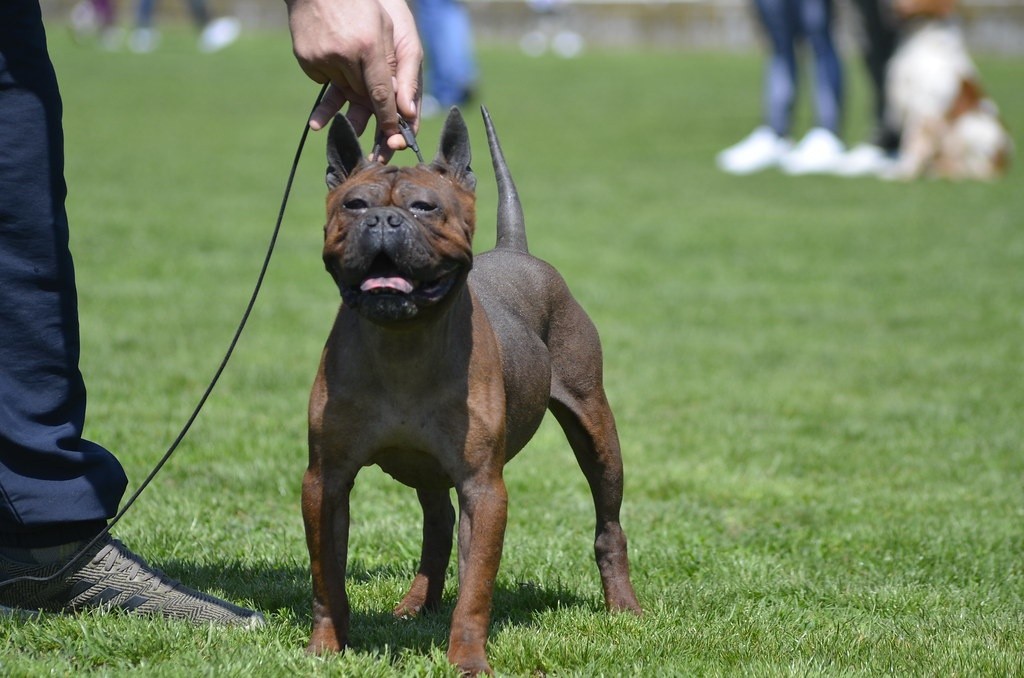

(0, 537), (267, 633)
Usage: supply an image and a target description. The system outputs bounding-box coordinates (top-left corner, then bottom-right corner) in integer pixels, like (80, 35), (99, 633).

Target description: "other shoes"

(200, 17), (240, 50)
(716, 126), (795, 175)
(844, 142), (887, 175)
(784, 128), (846, 177)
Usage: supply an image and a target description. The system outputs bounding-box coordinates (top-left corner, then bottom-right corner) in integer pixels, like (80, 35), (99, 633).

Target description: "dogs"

(878, 1), (1013, 183)
(304, 100), (644, 678)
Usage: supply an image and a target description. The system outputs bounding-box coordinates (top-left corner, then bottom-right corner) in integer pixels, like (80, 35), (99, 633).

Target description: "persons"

(845, 0), (958, 184)
(0, 0), (426, 639)
(714, 0), (844, 177)
(56, 0), (244, 51)
